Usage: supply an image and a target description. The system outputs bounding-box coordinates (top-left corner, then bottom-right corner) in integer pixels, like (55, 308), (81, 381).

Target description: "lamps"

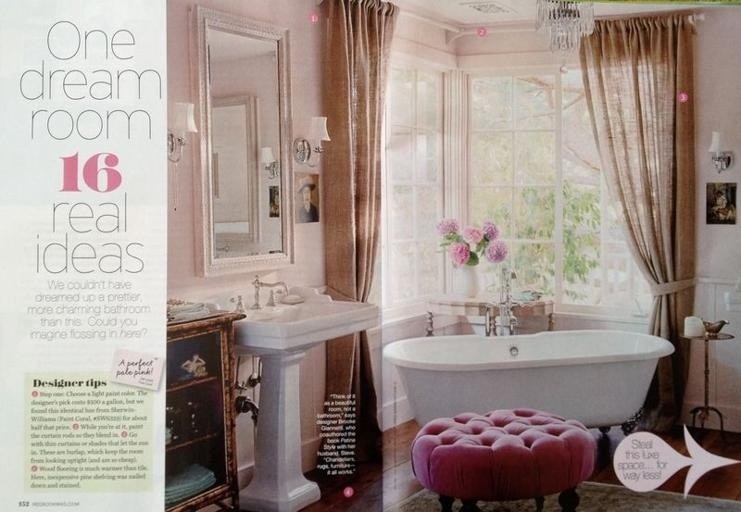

(262, 143), (282, 183)
(706, 131), (734, 175)
(533, 0), (604, 56)
(170, 102), (200, 166)
(295, 114), (336, 175)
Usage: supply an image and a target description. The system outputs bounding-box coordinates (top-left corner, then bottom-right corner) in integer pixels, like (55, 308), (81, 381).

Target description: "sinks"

(204, 284), (380, 357)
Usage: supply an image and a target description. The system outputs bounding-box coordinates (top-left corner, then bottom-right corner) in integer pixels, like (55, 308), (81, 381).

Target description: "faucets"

(251, 273), (289, 309)
(498, 267), (518, 337)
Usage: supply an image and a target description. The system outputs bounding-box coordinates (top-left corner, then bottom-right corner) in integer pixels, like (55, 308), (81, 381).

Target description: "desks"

(680, 331), (735, 442)
(419, 299), (557, 335)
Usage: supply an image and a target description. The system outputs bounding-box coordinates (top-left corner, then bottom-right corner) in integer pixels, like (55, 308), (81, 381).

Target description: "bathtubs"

(383, 329), (675, 428)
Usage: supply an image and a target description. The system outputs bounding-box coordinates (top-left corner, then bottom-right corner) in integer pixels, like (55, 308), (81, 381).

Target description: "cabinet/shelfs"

(166, 305), (254, 511)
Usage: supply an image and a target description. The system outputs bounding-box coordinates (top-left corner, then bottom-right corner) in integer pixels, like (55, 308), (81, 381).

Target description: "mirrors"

(188, 3), (301, 274)
(215, 94), (261, 252)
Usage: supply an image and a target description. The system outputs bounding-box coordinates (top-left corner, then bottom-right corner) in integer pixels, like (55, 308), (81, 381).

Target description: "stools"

(413, 406), (598, 512)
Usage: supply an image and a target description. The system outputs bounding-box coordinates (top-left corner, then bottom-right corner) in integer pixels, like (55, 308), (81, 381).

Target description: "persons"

(297, 174), (318, 222)
(707, 188), (731, 221)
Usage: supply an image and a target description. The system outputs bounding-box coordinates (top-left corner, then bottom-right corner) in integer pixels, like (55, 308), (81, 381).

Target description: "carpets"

(383, 483), (741, 512)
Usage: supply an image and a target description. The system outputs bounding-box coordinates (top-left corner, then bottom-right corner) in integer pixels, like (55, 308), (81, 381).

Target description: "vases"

(449, 261), (477, 297)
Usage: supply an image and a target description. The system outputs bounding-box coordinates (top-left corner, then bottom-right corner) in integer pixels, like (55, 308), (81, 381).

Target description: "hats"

(297, 176), (316, 193)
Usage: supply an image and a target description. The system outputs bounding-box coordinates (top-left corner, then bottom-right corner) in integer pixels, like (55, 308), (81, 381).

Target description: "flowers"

(437, 215), (507, 265)
